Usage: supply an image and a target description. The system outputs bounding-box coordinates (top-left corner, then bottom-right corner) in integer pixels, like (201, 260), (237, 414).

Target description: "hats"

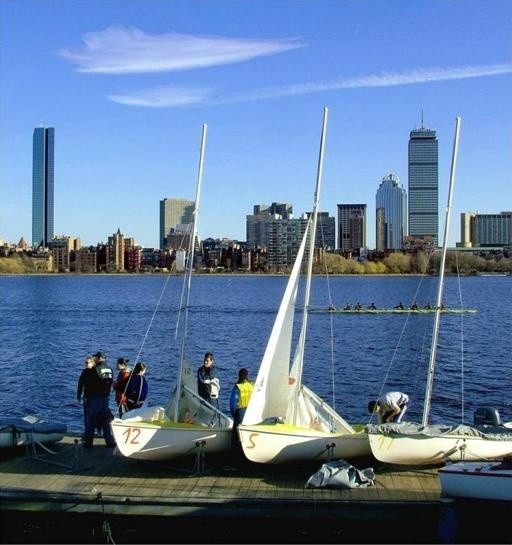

(92, 351), (107, 359)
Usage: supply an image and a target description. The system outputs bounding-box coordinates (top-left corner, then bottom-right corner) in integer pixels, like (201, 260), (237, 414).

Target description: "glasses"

(84, 362), (89, 364)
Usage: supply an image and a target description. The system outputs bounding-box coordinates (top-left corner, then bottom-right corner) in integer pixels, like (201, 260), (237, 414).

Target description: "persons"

(230, 368), (255, 454)
(198, 352), (220, 412)
(368, 391), (410, 424)
(77, 352), (148, 447)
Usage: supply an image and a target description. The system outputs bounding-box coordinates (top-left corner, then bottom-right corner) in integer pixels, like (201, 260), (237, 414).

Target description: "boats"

(0, 413), (71, 451)
(437, 458), (512, 503)
(329, 308), (477, 318)
